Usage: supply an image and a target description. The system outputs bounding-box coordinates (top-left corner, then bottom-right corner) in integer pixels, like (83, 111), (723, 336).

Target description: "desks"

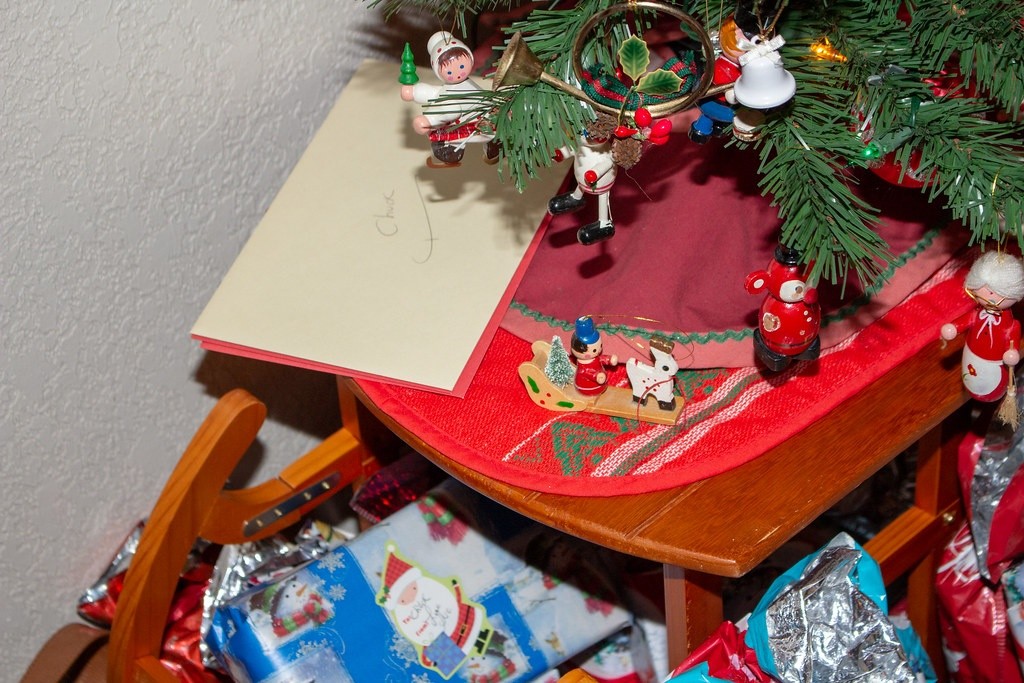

(104, 105), (1024, 683)
